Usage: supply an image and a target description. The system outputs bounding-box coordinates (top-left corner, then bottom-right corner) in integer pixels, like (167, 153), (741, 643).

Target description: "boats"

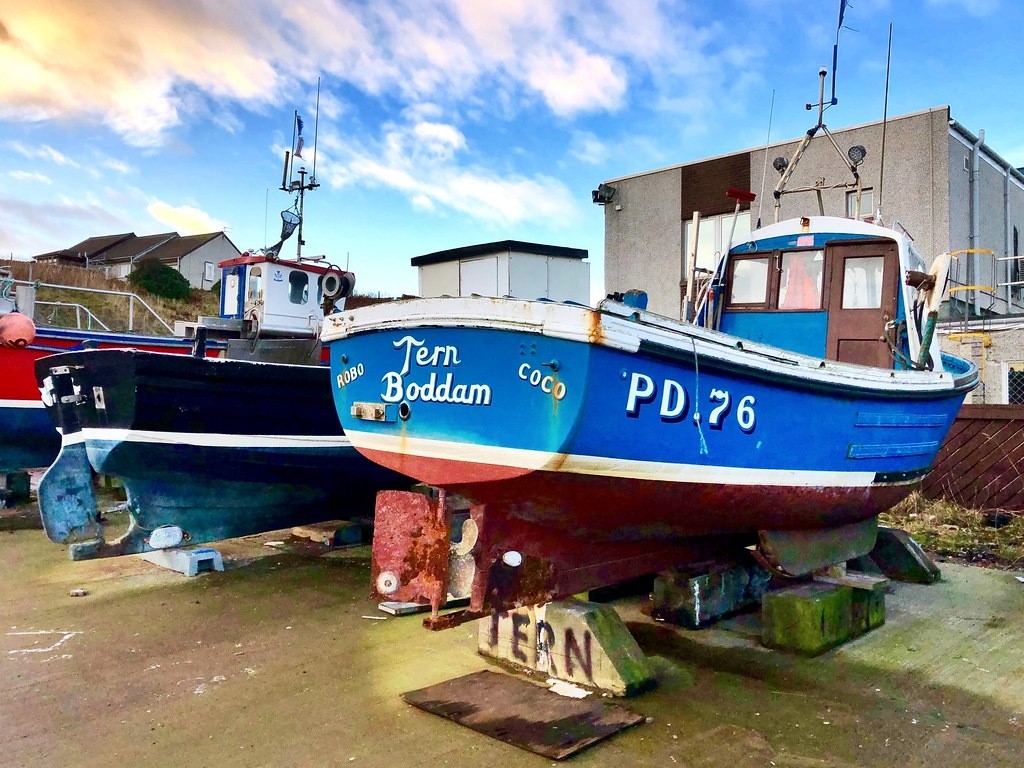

(0, 108), (426, 561)
(319, 0), (981, 631)
